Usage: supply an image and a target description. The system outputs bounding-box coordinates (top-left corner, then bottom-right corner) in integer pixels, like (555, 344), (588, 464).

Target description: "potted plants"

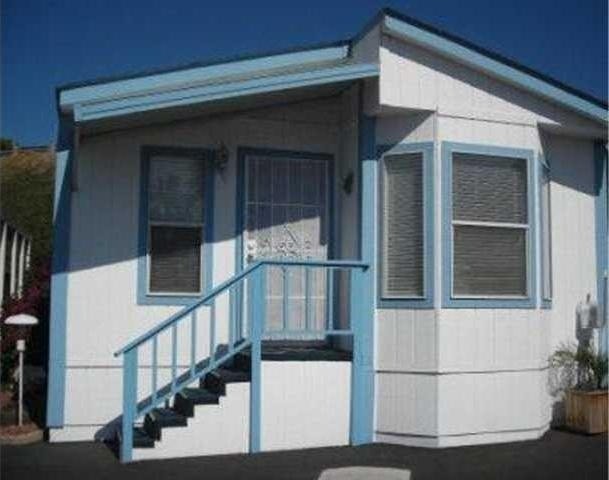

(552, 346), (608, 434)
